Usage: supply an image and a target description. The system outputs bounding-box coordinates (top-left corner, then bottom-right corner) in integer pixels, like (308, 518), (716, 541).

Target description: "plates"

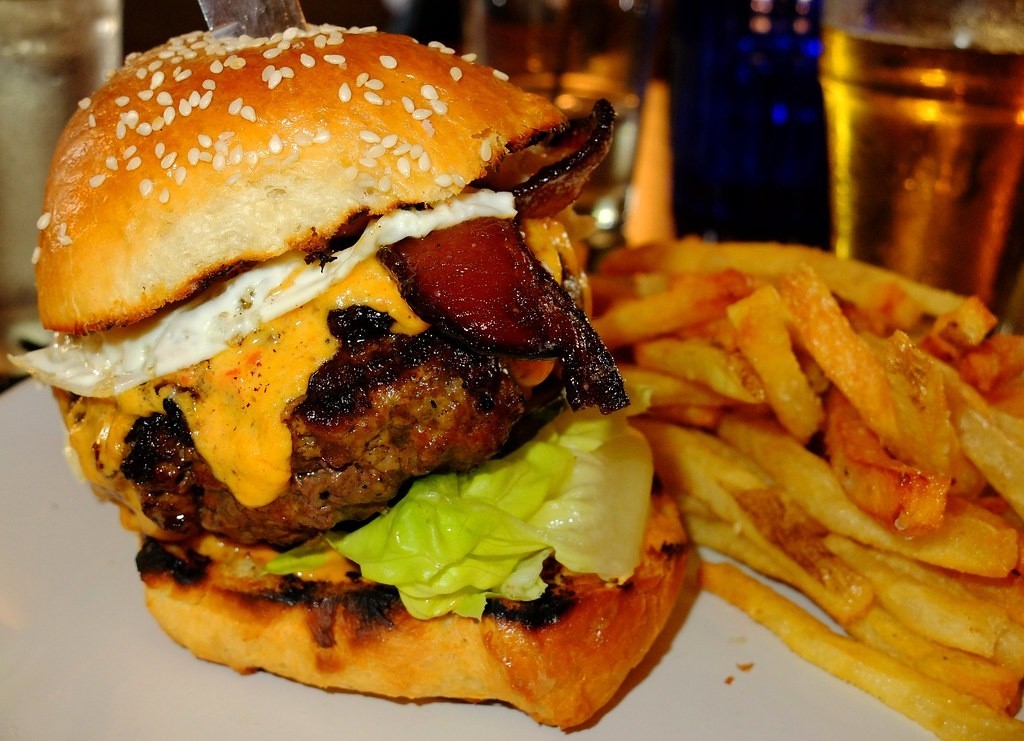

(0, 373), (945, 741)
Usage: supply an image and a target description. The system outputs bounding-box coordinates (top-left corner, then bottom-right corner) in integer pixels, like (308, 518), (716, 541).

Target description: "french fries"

(596, 239), (1024, 741)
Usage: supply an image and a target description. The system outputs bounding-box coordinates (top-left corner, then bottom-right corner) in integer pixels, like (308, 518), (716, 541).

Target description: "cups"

(816, 0), (1024, 340)
(0, 0), (123, 383)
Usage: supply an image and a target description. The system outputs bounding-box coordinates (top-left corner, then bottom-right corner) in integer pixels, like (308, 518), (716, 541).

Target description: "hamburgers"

(5, 18), (690, 729)
